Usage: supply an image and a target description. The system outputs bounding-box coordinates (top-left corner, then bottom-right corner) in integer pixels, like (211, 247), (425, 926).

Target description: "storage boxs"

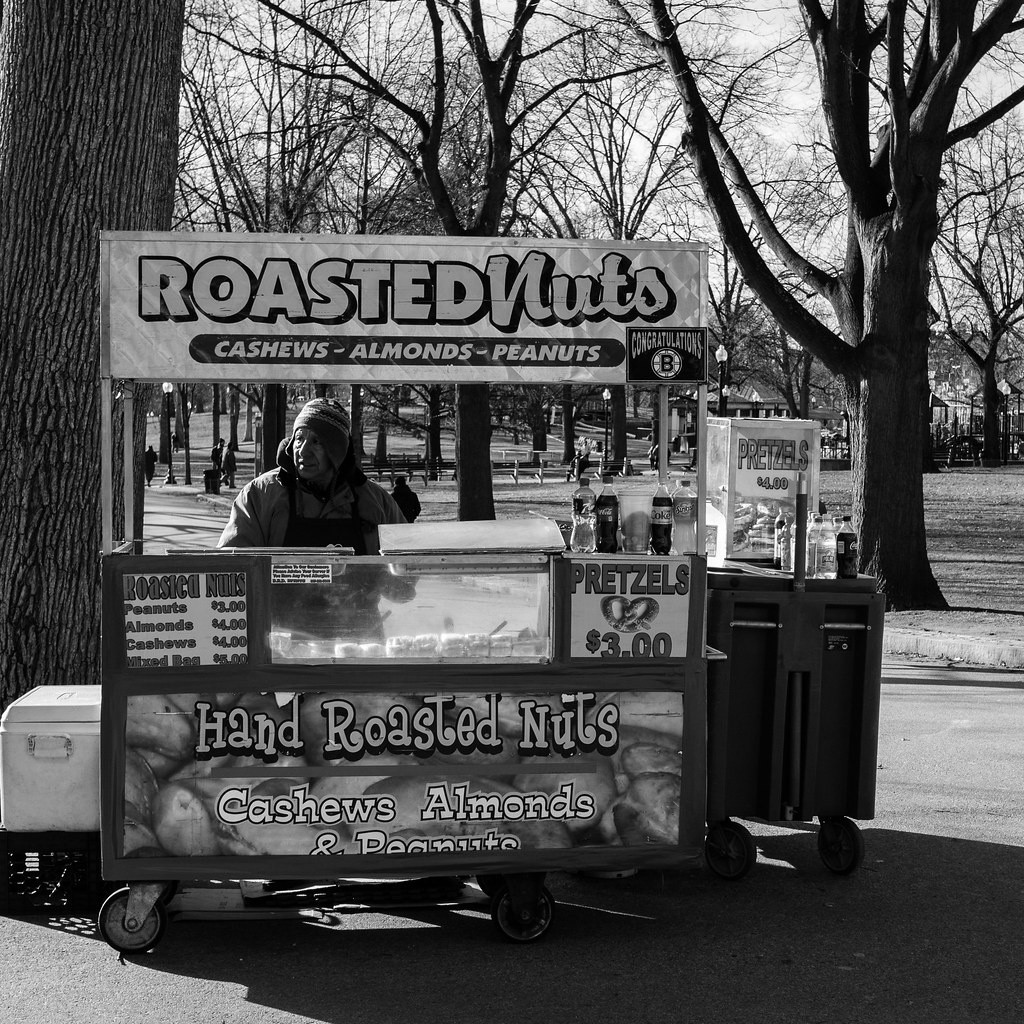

(0, 683), (128, 922)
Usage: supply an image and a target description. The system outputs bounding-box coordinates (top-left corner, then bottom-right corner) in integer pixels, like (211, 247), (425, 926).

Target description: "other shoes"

(230, 485), (236, 488)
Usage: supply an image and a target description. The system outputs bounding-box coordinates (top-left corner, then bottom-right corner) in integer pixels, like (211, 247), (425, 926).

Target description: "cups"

(618, 490), (654, 555)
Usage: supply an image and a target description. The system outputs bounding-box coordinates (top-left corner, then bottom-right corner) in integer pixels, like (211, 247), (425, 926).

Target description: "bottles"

(670, 480), (682, 555)
(570, 477), (596, 553)
(673, 480), (698, 554)
(772, 506), (858, 579)
(595, 476), (618, 553)
(651, 482), (672, 556)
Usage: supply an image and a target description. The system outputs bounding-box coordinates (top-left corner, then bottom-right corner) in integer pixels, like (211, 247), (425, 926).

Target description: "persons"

(647, 443), (671, 472)
(211, 439), (229, 486)
(391, 476), (421, 524)
(582, 441), (591, 459)
(219, 441), (237, 488)
(171, 432), (178, 453)
(291, 395), (298, 410)
(218, 398), (419, 643)
(145, 445), (158, 487)
(566, 450), (584, 481)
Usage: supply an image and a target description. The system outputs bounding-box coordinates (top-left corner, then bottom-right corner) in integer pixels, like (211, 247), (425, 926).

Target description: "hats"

(284, 397), (352, 471)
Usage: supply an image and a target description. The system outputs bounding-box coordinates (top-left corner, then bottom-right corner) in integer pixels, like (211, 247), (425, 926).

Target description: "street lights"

(1002, 382), (1011, 466)
(722, 385), (731, 417)
(162, 382), (177, 484)
(811, 397), (816, 408)
(602, 388), (612, 471)
(715, 344), (728, 417)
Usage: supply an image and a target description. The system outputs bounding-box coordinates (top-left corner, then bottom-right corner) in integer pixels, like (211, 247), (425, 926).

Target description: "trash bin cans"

(203, 469), (221, 495)
